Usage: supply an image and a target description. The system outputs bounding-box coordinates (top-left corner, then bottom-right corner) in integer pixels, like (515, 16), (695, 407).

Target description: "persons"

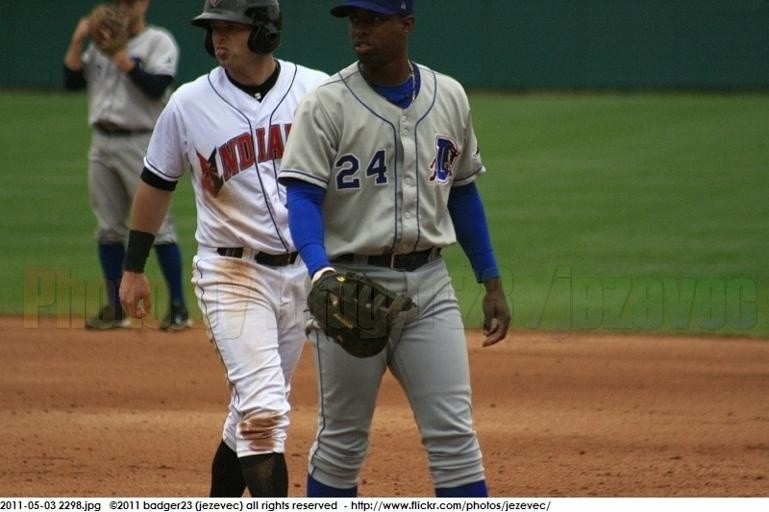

(118, 1), (331, 497)
(61, 1), (194, 332)
(276, 0), (511, 498)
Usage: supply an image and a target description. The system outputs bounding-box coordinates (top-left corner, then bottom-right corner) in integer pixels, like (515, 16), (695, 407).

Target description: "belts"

(328, 246), (442, 270)
(95, 119), (149, 136)
(218, 244), (298, 266)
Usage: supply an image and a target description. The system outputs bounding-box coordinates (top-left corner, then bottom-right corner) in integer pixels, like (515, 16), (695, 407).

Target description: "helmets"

(191, 2), (284, 54)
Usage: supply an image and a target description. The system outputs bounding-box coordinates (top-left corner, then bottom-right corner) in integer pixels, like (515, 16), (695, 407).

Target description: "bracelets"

(124, 230), (155, 273)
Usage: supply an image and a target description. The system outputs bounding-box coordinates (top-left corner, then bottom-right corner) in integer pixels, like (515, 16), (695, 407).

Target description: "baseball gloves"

(307, 270), (413, 358)
(87, 3), (129, 58)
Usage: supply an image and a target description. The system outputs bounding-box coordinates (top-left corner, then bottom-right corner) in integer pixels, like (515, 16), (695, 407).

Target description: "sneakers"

(161, 308), (194, 331)
(85, 306), (131, 331)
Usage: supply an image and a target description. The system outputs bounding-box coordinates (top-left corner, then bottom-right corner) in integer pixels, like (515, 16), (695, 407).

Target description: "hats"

(330, 1), (412, 18)
